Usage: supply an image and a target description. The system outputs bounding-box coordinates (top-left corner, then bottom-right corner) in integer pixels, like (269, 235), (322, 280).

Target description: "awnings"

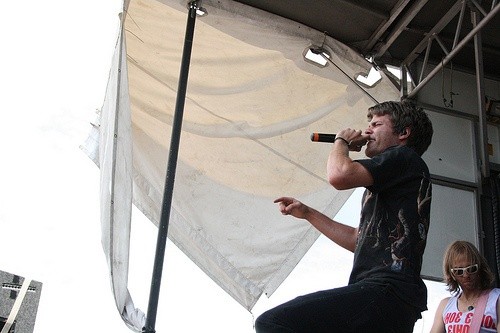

(78, 1), (413, 332)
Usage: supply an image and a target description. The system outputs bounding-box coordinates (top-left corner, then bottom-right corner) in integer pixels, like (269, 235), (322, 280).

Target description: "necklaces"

(455, 285), (482, 310)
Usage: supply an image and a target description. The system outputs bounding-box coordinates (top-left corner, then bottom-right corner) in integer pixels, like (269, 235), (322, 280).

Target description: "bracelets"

(333, 136), (351, 149)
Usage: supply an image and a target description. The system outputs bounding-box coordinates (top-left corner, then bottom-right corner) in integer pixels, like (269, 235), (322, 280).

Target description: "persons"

(255, 101), (426, 332)
(428, 241), (500, 333)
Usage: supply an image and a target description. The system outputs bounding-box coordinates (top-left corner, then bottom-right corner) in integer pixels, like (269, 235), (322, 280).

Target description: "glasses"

(451, 263), (479, 277)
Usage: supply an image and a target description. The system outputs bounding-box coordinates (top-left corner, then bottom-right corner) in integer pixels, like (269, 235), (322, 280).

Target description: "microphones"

(311, 133), (367, 148)
